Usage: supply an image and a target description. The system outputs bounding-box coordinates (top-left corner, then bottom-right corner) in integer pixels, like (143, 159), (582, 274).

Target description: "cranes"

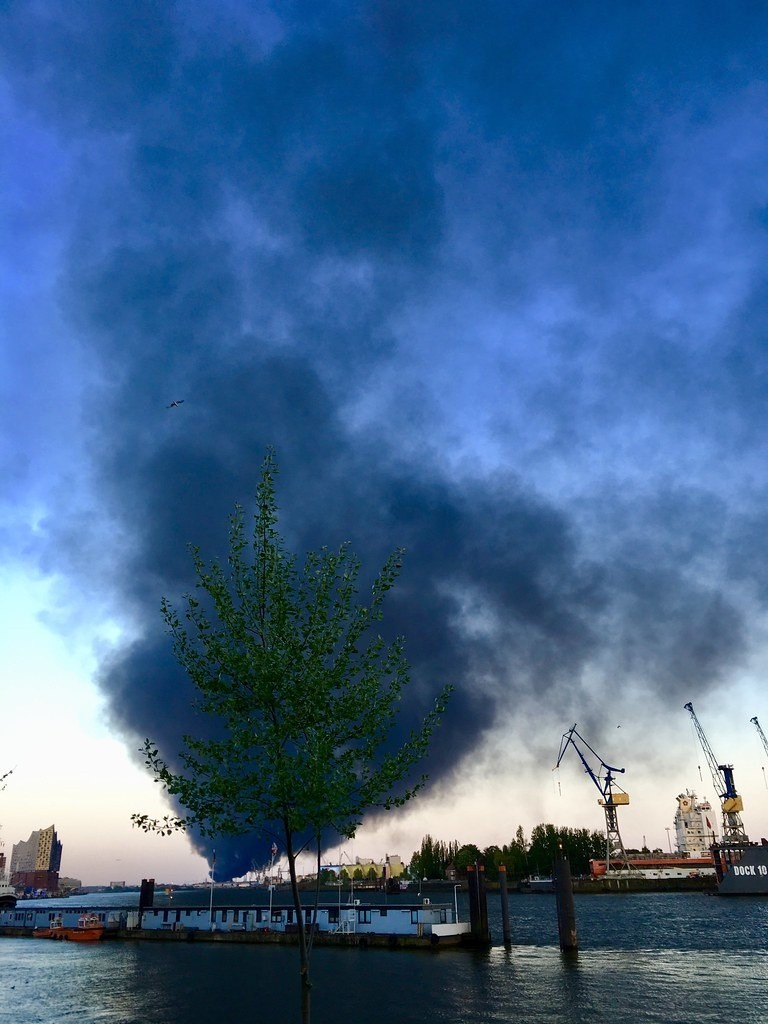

(555, 722), (646, 880)
(684, 701), (749, 863)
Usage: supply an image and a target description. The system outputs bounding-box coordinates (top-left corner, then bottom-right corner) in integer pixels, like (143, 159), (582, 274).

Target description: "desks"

(160, 922), (175, 932)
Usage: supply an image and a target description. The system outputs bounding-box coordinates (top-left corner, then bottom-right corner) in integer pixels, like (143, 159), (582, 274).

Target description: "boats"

(32, 923), (107, 943)
